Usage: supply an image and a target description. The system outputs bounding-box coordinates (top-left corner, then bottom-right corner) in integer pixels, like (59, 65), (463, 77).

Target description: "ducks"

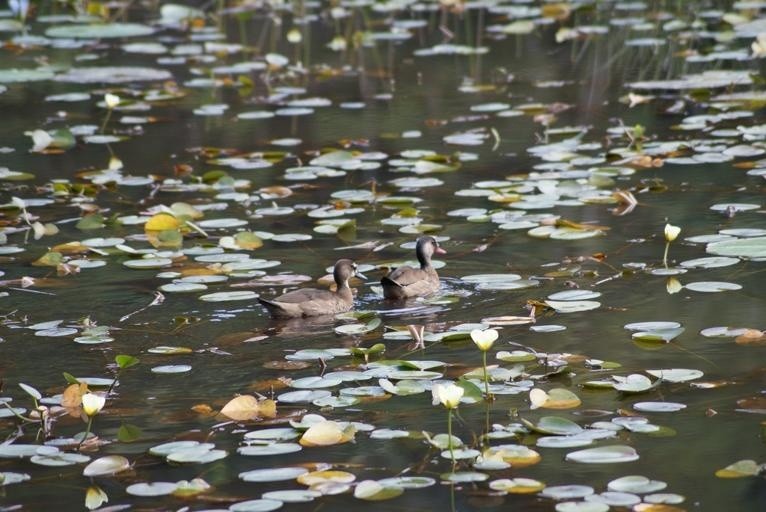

(381, 237), (440, 299)
(257, 258), (368, 319)
